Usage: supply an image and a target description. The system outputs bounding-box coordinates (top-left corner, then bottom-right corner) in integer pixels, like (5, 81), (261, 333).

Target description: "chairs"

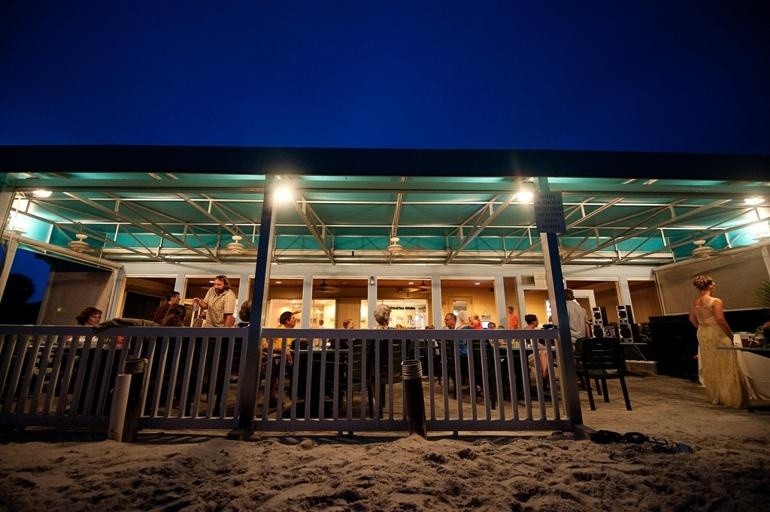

(277, 321), (632, 417)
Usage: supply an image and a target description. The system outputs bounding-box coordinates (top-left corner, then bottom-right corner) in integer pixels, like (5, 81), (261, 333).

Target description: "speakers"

(590, 304), (639, 343)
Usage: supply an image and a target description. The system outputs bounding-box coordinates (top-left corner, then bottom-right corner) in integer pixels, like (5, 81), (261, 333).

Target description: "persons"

(342, 318), (355, 329)
(75, 306), (102, 326)
(233, 300), (254, 328)
(689, 274), (746, 409)
(274, 311), (297, 366)
(374, 303), (396, 330)
(194, 276), (236, 328)
(161, 304), (187, 325)
(318, 320), (326, 329)
(425, 288), (591, 391)
(154, 291), (180, 322)
